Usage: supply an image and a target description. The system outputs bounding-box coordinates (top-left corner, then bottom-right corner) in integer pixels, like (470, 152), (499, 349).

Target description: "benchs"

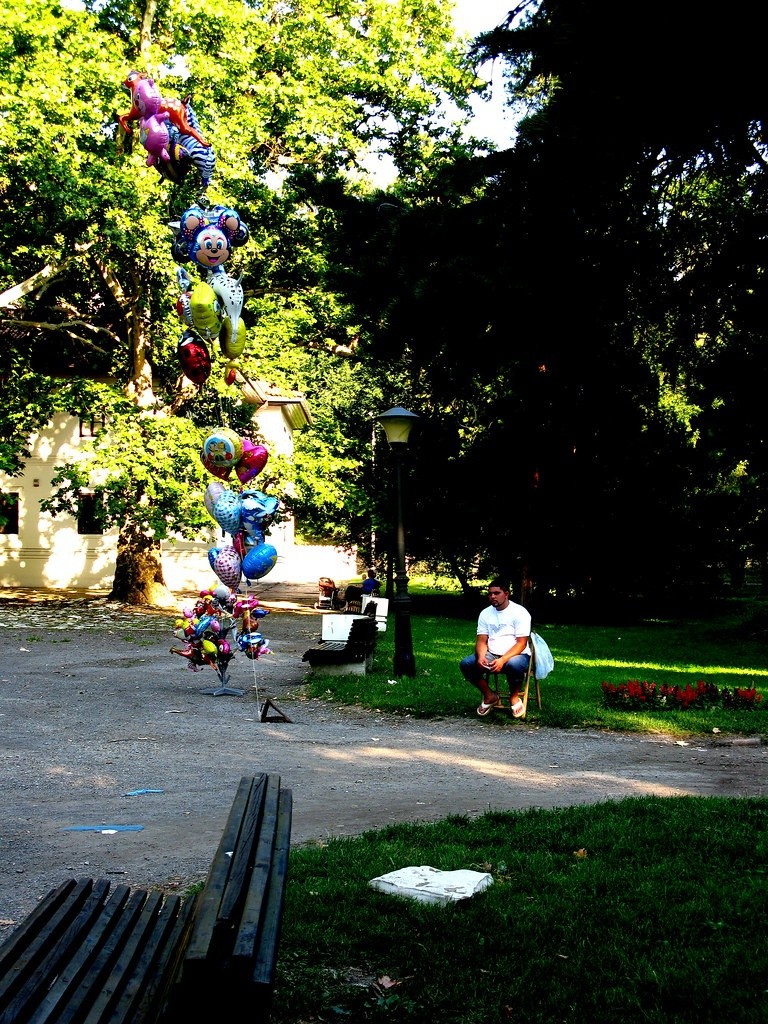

(0, 772), (294, 1024)
(323, 588), (380, 615)
(302, 616), (380, 675)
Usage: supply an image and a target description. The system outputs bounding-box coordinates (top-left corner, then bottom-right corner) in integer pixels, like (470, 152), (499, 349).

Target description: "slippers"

(511, 698), (525, 718)
(477, 695), (499, 715)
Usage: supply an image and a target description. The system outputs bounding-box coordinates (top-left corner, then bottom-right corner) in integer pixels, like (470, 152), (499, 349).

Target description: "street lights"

(373, 405), (421, 680)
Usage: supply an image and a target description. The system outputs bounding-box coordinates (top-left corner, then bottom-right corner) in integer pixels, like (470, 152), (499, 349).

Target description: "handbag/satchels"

(531, 631), (554, 680)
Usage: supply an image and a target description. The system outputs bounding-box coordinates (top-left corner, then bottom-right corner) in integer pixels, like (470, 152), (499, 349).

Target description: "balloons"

(119, 69), (279, 672)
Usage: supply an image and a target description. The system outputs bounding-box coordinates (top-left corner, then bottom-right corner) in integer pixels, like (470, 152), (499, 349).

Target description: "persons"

(459, 579), (531, 717)
(340, 570), (381, 611)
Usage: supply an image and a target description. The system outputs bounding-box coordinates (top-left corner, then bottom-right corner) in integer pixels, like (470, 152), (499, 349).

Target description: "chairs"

(481, 630), (555, 720)
